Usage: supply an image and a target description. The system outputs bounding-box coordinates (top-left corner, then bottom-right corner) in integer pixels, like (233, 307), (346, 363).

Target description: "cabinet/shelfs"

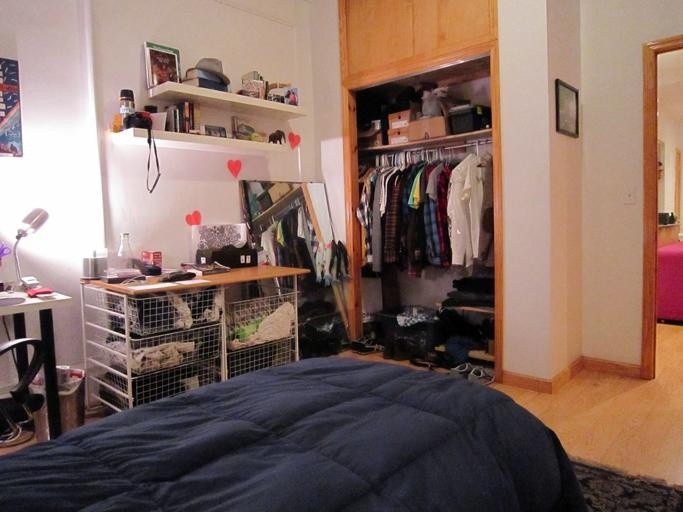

(336, 0), (504, 384)
(79, 257), (226, 416)
(110, 79), (309, 155)
(223, 264), (312, 381)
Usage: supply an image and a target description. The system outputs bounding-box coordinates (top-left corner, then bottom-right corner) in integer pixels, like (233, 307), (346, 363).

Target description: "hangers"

(356, 137), (490, 185)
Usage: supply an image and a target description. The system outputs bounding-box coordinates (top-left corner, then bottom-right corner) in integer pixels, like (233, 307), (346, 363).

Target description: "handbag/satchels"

(472, 260), (494, 286)
(306, 314), (344, 344)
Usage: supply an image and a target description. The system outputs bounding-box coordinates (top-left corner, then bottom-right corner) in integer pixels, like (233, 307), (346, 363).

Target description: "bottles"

(120, 89), (135, 123)
(118, 233), (133, 282)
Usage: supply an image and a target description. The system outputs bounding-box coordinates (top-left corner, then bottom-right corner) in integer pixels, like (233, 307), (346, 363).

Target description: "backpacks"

(357, 82), (410, 144)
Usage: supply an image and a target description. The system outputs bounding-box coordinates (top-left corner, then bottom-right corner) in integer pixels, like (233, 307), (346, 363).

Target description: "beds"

(0, 354), (585, 512)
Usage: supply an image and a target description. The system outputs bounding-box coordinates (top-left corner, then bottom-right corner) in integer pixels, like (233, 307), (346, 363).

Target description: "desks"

(0, 286), (74, 441)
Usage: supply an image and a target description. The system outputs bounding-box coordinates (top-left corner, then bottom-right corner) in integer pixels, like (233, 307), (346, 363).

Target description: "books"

(181, 78), (227, 92)
(186, 69), (222, 84)
(166, 101), (200, 134)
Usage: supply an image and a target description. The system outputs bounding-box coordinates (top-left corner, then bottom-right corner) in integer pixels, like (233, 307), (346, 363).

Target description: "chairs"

(0, 335), (48, 451)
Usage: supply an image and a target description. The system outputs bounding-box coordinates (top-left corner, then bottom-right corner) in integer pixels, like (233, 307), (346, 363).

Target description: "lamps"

(11, 206), (50, 291)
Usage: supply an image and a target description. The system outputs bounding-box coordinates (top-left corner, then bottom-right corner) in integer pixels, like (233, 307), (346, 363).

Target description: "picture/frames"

(555, 78), (580, 138)
(144, 40), (182, 88)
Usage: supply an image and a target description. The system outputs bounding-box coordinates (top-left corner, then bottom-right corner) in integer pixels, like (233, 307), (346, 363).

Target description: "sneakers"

(425, 352), (439, 367)
(449, 363), (483, 380)
(352, 335), (371, 352)
(398, 343), (423, 359)
(468, 366), (494, 385)
(358, 339), (378, 355)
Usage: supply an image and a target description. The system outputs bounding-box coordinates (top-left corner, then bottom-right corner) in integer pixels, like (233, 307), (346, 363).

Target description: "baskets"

(229, 339), (292, 378)
(94, 322), (223, 376)
(95, 288), (221, 337)
(94, 358), (221, 410)
(225, 283), (300, 352)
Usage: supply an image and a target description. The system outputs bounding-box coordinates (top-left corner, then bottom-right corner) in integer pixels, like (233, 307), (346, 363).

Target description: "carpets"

(567, 454), (683, 512)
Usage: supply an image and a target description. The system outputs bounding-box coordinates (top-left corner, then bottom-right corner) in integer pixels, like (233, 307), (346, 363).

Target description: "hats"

(186, 58), (230, 85)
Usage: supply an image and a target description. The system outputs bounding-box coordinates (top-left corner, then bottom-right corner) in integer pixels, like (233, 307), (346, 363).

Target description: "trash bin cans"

(28, 365), (86, 444)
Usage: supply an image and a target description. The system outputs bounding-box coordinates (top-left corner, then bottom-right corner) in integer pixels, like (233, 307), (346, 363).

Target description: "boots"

(393, 337), (405, 361)
(383, 335), (394, 359)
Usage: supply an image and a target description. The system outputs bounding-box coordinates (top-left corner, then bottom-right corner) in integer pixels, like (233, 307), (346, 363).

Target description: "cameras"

(124, 112), (152, 128)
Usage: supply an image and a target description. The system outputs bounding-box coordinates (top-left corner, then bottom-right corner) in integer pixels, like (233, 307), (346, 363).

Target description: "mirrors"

(657, 137), (667, 214)
(252, 196), (302, 233)
(239, 177), (350, 356)
